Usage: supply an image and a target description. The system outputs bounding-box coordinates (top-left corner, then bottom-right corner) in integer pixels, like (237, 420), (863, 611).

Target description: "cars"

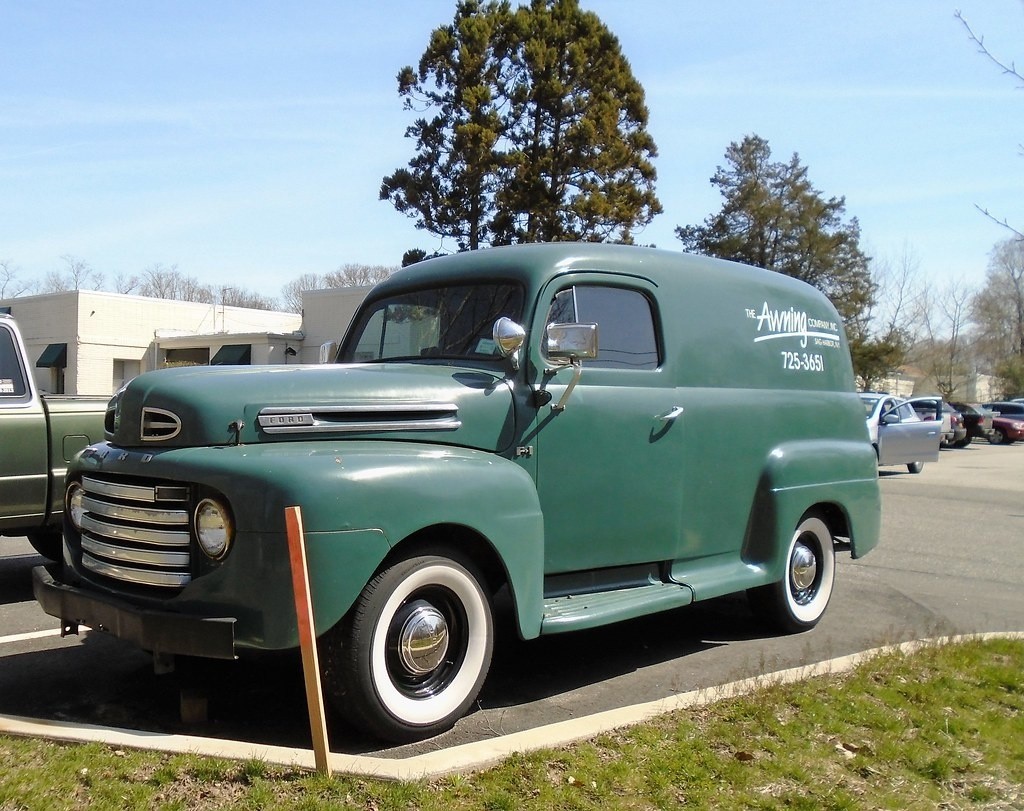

(901, 397), (1024, 449)
(28, 242), (883, 746)
(857, 392), (942, 474)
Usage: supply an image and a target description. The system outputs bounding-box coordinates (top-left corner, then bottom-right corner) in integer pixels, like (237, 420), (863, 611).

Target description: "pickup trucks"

(1, 313), (113, 566)
